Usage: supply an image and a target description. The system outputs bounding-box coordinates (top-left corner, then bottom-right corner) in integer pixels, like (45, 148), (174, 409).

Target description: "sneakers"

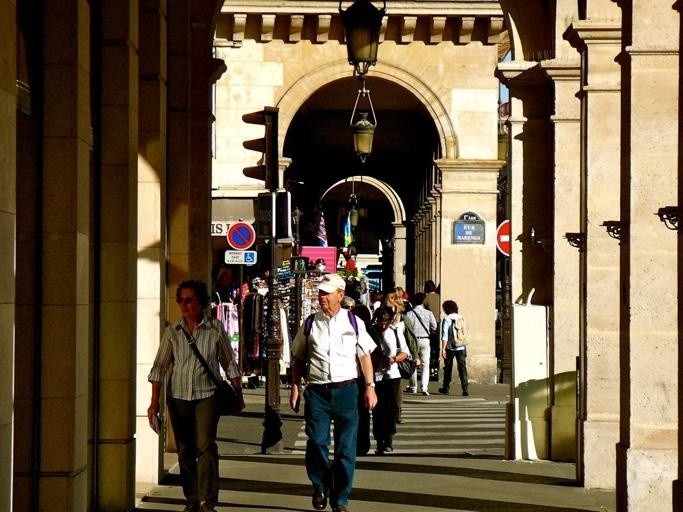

(355, 439), (393, 456)
(405, 386), (468, 396)
(182, 502), (216, 512)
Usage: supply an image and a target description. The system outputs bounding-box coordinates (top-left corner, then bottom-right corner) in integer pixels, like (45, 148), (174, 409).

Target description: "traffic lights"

(239, 106), (279, 192)
(291, 256), (309, 273)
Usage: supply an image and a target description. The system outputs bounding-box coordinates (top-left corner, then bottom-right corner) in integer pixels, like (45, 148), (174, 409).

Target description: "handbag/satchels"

(397, 353), (416, 379)
(429, 335), (437, 348)
(214, 380), (241, 417)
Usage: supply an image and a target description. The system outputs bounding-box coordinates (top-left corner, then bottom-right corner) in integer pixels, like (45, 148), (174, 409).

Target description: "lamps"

(335, 0), (390, 75)
(342, 76), (380, 167)
(347, 180), (359, 232)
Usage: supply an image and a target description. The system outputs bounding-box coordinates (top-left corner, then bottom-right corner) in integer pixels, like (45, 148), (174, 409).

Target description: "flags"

(303, 203), (330, 246)
(343, 210), (355, 249)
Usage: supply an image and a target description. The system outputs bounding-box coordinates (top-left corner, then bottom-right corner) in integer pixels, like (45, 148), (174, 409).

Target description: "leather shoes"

(311, 489), (328, 511)
(331, 506), (347, 512)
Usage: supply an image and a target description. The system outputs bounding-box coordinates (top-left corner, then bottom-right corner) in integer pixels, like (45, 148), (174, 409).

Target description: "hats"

(315, 275), (347, 295)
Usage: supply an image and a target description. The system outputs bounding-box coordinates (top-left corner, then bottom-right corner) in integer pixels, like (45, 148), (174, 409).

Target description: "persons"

(367, 280), (472, 397)
(343, 280), (419, 455)
(290, 275), (379, 512)
(148, 277), (246, 511)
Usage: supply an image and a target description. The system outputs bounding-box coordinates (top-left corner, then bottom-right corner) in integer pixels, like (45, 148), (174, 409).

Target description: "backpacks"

(451, 317), (469, 348)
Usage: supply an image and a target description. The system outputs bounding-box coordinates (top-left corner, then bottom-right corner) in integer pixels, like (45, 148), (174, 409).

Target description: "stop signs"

(496, 220), (511, 257)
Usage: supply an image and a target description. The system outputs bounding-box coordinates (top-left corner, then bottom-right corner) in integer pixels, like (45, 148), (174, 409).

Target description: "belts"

(326, 379), (355, 388)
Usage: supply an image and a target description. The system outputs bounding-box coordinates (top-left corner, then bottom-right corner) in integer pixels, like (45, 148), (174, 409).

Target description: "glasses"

(176, 296), (193, 304)
